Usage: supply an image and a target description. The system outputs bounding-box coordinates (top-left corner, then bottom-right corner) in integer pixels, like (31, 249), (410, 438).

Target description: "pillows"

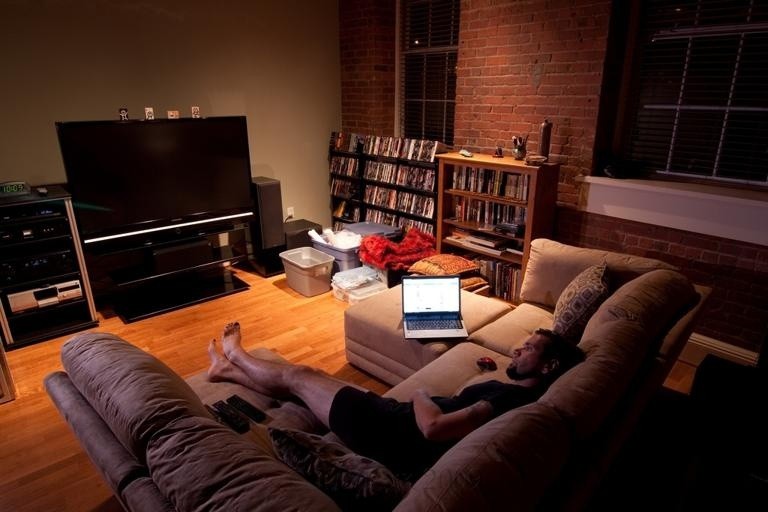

(460, 276), (489, 290)
(553, 258), (609, 340)
(265, 427), (402, 511)
(407, 252), (480, 278)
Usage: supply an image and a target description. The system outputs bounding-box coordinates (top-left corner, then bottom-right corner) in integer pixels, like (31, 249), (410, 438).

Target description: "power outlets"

(287, 207), (295, 219)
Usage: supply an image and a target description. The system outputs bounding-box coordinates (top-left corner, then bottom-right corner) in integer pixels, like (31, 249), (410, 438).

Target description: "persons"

(206, 322), (564, 466)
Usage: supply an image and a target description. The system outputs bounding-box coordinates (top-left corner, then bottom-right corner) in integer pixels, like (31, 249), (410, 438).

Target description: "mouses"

(476, 355), (497, 372)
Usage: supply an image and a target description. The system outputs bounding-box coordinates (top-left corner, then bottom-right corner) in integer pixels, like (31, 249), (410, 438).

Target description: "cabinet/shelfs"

(435, 151), (559, 303)
(0, 182), (98, 348)
(326, 133), (448, 238)
(81, 209), (255, 324)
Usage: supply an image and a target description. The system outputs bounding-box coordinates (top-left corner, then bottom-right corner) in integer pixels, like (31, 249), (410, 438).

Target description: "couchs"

(42, 330), (296, 507)
(123, 318), (649, 511)
(468, 237), (712, 363)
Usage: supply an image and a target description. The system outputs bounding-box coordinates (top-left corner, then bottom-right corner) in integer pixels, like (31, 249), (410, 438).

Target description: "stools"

(285, 219), (322, 248)
(344, 271), (512, 387)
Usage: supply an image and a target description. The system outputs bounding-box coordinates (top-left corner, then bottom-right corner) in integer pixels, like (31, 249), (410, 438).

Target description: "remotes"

(459, 148), (473, 157)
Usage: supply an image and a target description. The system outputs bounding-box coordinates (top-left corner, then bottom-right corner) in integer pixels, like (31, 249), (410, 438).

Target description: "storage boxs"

(311, 231), (363, 272)
(343, 220), (403, 243)
(278, 246), (335, 297)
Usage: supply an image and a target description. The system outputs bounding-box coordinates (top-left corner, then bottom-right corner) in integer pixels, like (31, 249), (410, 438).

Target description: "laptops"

(400, 274), (470, 340)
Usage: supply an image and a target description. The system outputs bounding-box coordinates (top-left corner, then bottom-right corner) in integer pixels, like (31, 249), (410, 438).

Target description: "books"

(446, 227), (524, 257)
(452, 165), (528, 201)
(451, 195), (527, 222)
(464, 254), (521, 302)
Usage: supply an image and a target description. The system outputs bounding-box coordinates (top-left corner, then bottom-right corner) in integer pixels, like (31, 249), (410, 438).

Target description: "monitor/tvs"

(54, 115), (254, 246)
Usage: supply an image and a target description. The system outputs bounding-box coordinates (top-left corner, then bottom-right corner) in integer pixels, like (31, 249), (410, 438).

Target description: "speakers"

(250, 176), (286, 250)
